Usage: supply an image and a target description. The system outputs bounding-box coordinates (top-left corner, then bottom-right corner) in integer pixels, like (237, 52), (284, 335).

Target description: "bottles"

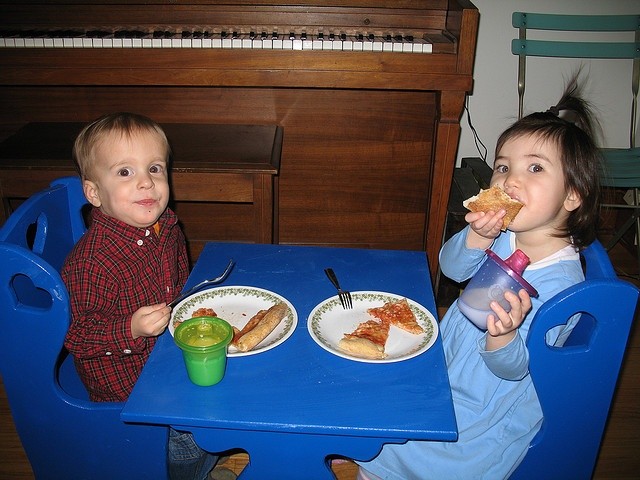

(457, 249), (539, 332)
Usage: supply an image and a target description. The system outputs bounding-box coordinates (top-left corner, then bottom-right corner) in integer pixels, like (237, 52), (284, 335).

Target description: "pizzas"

(232, 304), (288, 352)
(367, 298), (426, 336)
(192, 308), (218, 317)
(338, 320), (391, 359)
(463, 186), (523, 233)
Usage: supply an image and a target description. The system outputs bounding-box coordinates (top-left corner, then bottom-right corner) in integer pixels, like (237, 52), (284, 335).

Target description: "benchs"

(0, 121), (284, 242)
(509, 13), (640, 245)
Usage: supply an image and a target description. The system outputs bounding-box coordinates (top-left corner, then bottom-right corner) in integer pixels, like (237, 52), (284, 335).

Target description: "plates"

(306, 290), (439, 364)
(168, 286), (298, 358)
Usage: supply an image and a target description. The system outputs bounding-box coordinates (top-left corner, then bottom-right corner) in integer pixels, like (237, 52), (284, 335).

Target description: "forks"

(165, 261), (233, 308)
(324, 268), (353, 309)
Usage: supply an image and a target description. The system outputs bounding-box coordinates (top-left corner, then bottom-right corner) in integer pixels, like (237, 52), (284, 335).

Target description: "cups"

(173, 317), (233, 387)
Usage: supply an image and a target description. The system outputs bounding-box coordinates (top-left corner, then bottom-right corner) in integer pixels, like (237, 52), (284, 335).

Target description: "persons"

(58, 111), (236, 480)
(354, 61), (606, 479)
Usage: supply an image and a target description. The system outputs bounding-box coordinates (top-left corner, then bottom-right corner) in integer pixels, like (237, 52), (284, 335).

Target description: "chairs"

(507, 240), (640, 480)
(0, 177), (169, 480)
